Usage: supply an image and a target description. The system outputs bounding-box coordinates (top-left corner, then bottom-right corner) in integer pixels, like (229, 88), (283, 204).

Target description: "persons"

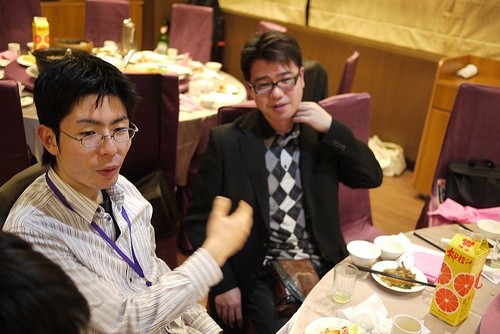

(0, 229), (91, 334)
(3, 51), (252, 334)
(187, 30), (384, 334)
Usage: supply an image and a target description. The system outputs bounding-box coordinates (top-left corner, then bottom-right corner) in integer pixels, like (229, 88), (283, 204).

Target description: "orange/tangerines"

(434, 261), (474, 314)
(33, 34), (50, 50)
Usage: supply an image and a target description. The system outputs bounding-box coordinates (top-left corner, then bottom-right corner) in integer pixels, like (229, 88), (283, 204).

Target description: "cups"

(333, 263), (359, 304)
(8, 41), (223, 108)
(490, 238), (500, 269)
(390, 314), (423, 334)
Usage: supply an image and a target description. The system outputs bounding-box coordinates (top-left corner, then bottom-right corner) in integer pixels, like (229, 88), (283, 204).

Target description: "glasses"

(59, 121), (139, 148)
(247, 70), (299, 95)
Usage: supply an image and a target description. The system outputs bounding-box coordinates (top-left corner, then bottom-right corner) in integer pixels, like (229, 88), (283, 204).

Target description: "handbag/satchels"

(368, 135), (406, 177)
(131, 170), (182, 240)
(443, 156), (500, 209)
(263, 257), (319, 316)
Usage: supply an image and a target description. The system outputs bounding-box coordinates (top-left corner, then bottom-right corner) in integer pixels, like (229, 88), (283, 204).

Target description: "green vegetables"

(401, 284), (411, 289)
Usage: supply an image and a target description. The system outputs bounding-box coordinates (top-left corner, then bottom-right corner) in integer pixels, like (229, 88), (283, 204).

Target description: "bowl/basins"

(347, 240), (381, 269)
(55, 39), (94, 54)
(31, 48), (91, 73)
(374, 235), (407, 261)
(477, 219), (500, 241)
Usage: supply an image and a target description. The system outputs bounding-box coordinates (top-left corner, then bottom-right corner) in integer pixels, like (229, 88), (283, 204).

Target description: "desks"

(21, 50), (246, 201)
(266, 210), (500, 334)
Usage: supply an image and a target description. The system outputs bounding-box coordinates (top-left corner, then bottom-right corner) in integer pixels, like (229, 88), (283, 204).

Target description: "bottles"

(123, 18), (138, 53)
(155, 19), (169, 55)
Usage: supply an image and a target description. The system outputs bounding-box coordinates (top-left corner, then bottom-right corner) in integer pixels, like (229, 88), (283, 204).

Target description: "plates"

(16, 54), (35, 66)
(25, 66), (39, 78)
(371, 260), (428, 293)
(486, 238), (500, 259)
(124, 50), (174, 63)
(125, 61), (192, 81)
(304, 317), (368, 334)
(0, 69), (5, 80)
(21, 95), (33, 108)
(0, 58), (10, 67)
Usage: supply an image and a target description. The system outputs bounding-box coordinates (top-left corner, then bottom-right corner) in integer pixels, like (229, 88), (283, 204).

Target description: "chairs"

(0, 0), (500, 290)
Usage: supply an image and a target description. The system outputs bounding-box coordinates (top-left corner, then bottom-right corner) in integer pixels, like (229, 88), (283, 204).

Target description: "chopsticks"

(348, 220), (494, 287)
